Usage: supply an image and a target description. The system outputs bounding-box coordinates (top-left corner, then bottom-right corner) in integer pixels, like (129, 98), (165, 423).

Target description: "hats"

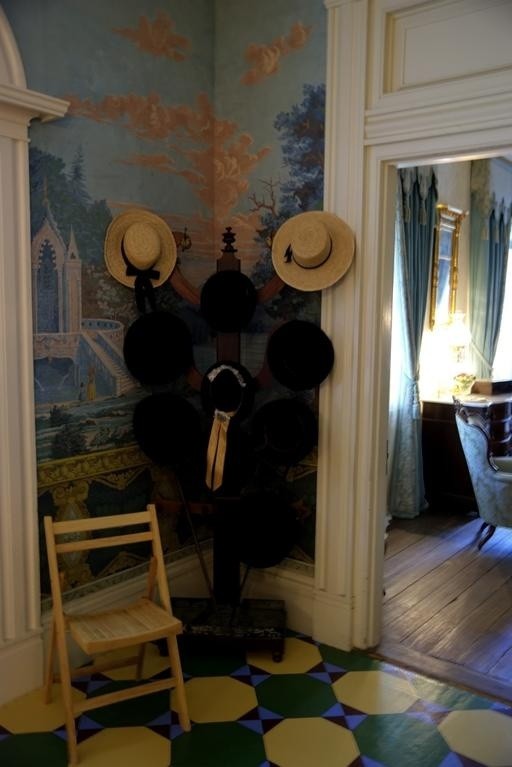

(104, 209), (178, 289)
(271, 210), (355, 292)
(200, 360), (256, 424)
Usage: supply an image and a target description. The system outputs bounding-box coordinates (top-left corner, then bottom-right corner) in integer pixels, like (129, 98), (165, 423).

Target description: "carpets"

(1, 629), (511, 766)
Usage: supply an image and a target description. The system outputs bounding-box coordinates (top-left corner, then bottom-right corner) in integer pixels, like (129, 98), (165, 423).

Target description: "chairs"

(42, 504), (192, 767)
(454, 406), (512, 550)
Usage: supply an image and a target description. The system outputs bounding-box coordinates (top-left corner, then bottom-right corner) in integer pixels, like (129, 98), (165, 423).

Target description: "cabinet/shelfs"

(419, 392), (512, 518)
